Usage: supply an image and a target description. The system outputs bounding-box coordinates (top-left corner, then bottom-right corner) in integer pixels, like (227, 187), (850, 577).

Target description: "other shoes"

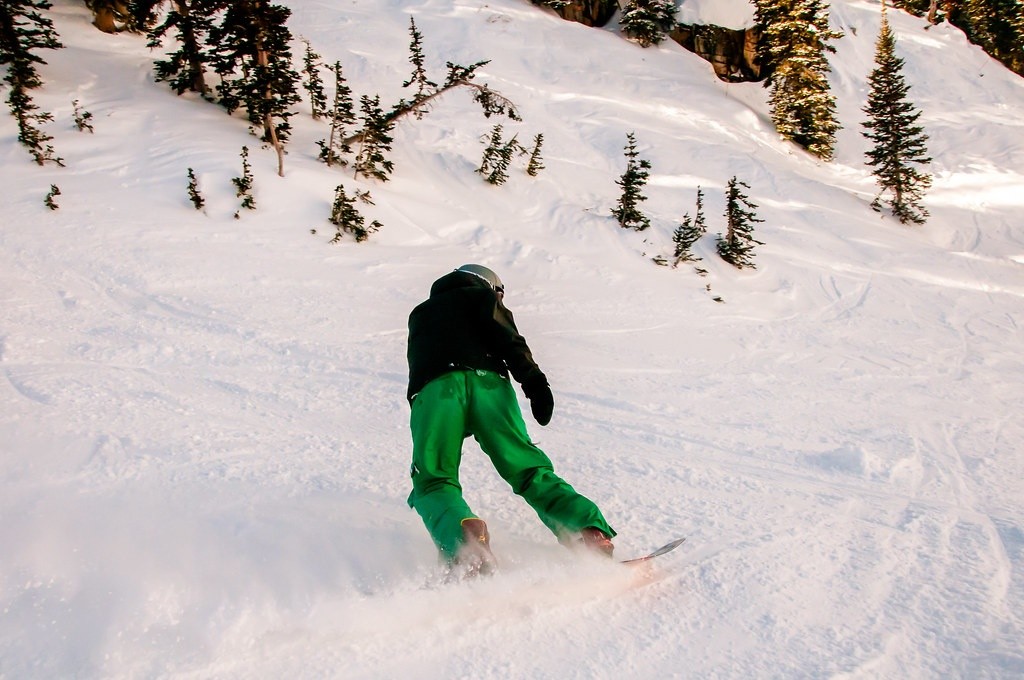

(584, 530), (613, 560)
(441, 518), (499, 584)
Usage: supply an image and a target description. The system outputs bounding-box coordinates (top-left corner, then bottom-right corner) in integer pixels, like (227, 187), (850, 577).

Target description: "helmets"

(458, 264), (504, 294)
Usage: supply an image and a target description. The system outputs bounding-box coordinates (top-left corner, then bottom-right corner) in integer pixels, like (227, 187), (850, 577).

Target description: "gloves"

(529, 387), (555, 426)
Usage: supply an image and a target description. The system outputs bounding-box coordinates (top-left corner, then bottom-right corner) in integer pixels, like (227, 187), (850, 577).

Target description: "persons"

(407, 265), (617, 579)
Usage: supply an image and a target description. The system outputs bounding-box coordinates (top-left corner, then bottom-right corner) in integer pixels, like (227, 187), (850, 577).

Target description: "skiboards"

(424, 533), (685, 588)
